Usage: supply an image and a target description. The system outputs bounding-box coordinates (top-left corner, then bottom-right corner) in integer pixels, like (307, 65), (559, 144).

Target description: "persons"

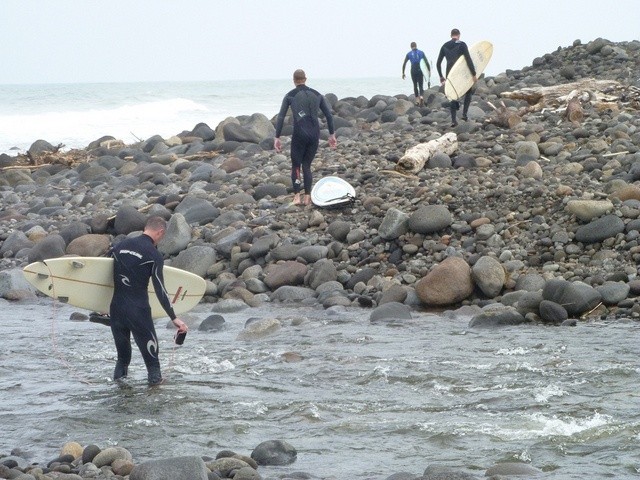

(402, 41), (431, 106)
(274, 68), (337, 205)
(109, 216), (189, 385)
(436, 28), (477, 127)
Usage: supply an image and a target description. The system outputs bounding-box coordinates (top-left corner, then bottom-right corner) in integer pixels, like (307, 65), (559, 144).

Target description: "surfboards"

(23, 257), (207, 320)
(311, 177), (354, 208)
(420, 58), (434, 88)
(445, 41), (494, 101)
